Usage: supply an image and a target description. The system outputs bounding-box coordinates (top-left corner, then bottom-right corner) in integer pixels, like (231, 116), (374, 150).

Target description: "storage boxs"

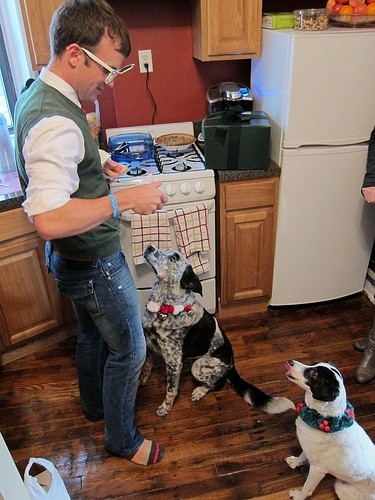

(203, 111), (272, 170)
(262, 11), (295, 30)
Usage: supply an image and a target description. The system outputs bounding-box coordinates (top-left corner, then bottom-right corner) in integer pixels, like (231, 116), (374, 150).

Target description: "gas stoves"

(104, 121), (216, 206)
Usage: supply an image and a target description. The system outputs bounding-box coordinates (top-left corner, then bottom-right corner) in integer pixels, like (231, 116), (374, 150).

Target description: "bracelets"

(108, 194), (118, 217)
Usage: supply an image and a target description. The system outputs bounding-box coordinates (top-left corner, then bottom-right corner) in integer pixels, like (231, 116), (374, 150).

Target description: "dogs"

(139, 244), (297, 417)
(285, 359), (375, 500)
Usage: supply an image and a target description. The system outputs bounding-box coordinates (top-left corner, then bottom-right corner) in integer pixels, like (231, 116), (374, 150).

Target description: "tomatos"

(326, 0), (375, 23)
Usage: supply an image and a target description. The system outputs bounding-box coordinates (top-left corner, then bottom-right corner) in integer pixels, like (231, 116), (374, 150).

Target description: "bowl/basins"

(293, 8), (330, 31)
(330, 13), (375, 28)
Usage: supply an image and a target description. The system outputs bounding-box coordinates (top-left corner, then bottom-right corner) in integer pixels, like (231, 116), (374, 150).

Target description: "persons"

(13, 0), (169, 465)
(353, 125), (375, 382)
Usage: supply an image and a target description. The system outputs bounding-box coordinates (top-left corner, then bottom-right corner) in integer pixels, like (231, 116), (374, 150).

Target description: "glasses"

(81, 45), (134, 85)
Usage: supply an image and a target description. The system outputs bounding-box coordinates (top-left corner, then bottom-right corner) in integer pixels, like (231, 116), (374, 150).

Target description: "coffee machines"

(206, 82), (254, 115)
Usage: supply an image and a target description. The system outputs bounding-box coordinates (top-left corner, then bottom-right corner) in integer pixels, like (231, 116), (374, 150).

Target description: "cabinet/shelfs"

(190, 0), (263, 62)
(218, 177), (279, 320)
(0, 207), (73, 368)
(19, 0), (66, 71)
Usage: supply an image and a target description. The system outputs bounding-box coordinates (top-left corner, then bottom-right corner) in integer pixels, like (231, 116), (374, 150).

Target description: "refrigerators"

(250, 25), (375, 307)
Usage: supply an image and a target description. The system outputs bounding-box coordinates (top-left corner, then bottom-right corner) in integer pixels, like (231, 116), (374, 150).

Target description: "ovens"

(119, 198), (217, 324)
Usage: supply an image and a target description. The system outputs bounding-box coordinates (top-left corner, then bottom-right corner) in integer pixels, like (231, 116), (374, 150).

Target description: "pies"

(156, 134), (195, 147)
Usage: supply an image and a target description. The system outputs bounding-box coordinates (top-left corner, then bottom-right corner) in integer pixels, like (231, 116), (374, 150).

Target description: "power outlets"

(138, 49), (154, 74)
